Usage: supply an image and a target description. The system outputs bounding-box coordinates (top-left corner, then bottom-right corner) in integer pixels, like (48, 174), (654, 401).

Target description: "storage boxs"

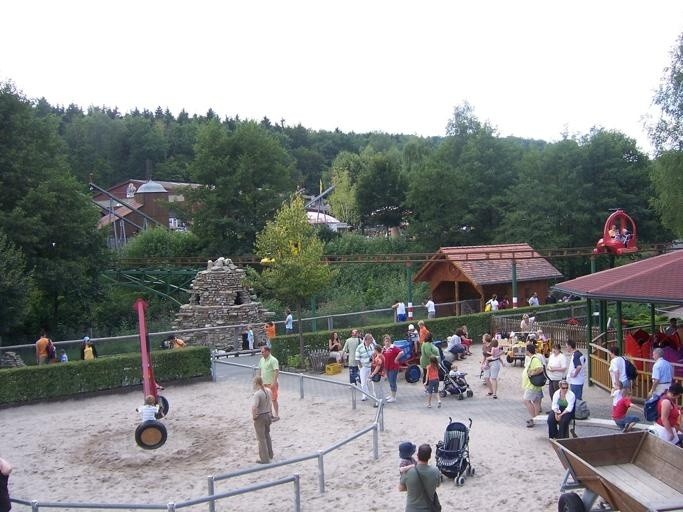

(325, 364), (343, 375)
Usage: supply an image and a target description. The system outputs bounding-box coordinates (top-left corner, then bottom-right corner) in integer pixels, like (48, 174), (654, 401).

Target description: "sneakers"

(362, 395), (396, 407)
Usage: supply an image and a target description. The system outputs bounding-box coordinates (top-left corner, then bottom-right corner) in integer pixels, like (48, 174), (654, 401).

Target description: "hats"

(419, 444), (430, 460)
(399, 442), (416, 459)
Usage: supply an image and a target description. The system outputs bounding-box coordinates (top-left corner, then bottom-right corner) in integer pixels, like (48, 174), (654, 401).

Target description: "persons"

(0, 458), (12, 512)
(400, 444), (442, 511)
(244, 309), (294, 422)
(519, 314), (683, 444)
(251, 377), (274, 464)
(398, 441), (417, 471)
(528, 290), (581, 307)
(167, 335), (187, 349)
(137, 395), (159, 422)
(608, 224), (631, 248)
(35, 328), (99, 366)
(327, 294), (507, 408)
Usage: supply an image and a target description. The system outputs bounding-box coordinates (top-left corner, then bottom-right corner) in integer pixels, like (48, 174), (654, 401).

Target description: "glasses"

(561, 386), (568, 390)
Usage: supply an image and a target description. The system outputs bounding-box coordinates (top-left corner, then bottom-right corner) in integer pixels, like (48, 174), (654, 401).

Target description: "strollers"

(435, 416), (475, 486)
(438, 359), (473, 400)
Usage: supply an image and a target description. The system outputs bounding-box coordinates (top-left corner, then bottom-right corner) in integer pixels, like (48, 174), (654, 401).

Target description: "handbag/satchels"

(575, 399), (590, 419)
(529, 373), (547, 386)
(372, 374), (380, 381)
(430, 492), (441, 512)
(625, 360), (637, 379)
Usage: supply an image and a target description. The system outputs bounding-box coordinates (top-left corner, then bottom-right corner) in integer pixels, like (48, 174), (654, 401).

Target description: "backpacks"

(644, 395), (660, 421)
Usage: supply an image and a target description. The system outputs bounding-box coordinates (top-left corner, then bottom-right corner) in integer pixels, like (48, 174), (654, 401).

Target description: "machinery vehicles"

(370, 332), (445, 382)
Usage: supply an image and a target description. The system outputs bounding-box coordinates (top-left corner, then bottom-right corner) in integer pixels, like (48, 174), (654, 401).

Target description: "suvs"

(506, 335), (551, 363)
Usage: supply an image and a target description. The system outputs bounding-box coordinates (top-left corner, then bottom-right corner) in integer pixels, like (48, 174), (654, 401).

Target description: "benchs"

(434, 348), (459, 366)
(327, 351), (348, 364)
(531, 414), (649, 437)
